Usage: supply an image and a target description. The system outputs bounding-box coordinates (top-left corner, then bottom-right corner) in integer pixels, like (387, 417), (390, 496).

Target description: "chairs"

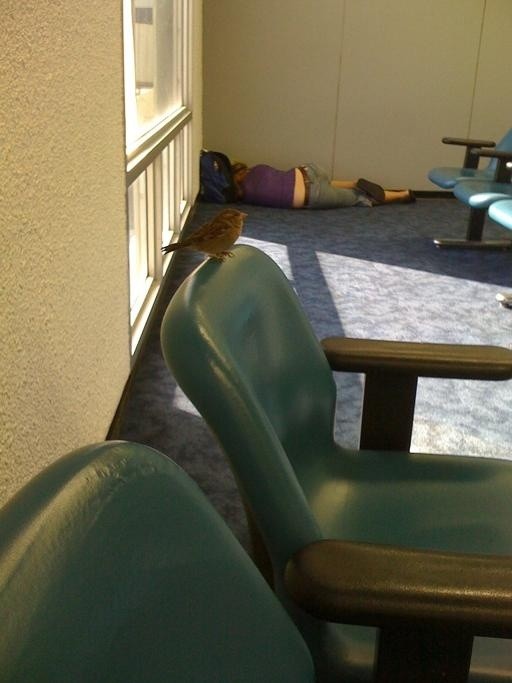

(160, 242), (512, 683)
(0, 438), (320, 683)
(424, 125), (511, 244)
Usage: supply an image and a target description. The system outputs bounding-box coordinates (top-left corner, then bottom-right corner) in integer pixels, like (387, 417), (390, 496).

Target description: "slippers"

(357, 178), (385, 203)
(408, 189), (415, 202)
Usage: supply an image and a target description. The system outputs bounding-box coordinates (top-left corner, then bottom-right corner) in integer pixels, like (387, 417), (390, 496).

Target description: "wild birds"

(160, 208), (248, 260)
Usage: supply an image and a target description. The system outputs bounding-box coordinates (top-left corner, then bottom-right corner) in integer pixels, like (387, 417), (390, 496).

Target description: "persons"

(232, 162), (417, 210)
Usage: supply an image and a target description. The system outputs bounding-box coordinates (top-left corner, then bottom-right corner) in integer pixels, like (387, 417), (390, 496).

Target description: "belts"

(298, 166), (310, 205)
